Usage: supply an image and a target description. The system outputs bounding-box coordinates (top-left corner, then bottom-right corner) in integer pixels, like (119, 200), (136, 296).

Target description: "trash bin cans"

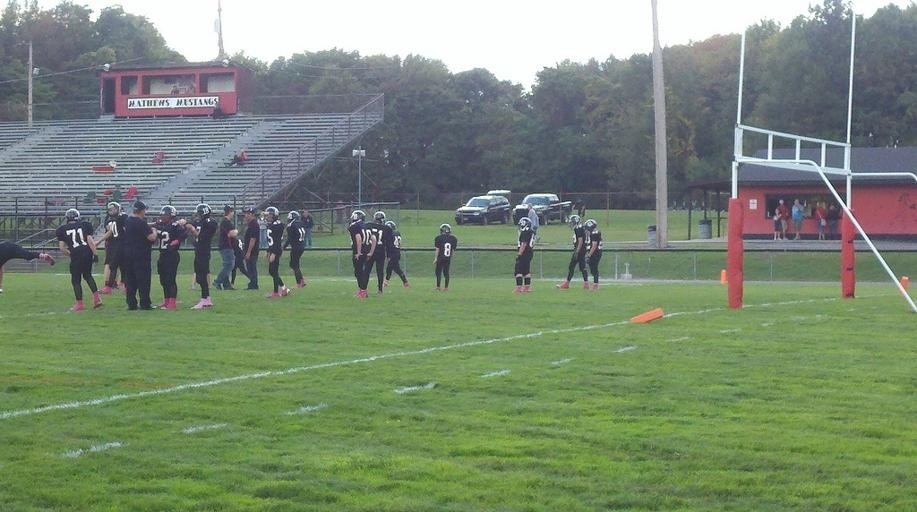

(648, 224), (656, 244)
(698, 219), (712, 239)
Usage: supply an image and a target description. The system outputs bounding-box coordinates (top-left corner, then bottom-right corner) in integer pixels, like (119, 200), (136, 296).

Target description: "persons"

(791, 198), (804, 240)
(777, 199), (790, 240)
(432, 224), (457, 292)
(773, 209), (782, 240)
(560, 214), (589, 289)
(816, 202), (827, 240)
(828, 203), (840, 240)
(585, 219), (603, 290)
(0, 239), (55, 293)
(572, 200), (585, 222)
(528, 203), (539, 241)
(511, 217), (535, 294)
(229, 150), (248, 168)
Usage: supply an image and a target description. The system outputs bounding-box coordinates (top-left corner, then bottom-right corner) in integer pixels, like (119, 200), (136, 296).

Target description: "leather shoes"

(128, 303), (159, 311)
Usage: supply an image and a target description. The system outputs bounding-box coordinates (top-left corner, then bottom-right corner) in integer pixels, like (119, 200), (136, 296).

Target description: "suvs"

(486, 188), (511, 201)
(452, 193), (512, 226)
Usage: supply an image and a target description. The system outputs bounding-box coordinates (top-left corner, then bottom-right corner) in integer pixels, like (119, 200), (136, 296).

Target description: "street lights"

(352, 145), (366, 211)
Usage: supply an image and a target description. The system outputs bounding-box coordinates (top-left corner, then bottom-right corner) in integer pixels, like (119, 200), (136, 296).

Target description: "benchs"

(0, 114), (381, 217)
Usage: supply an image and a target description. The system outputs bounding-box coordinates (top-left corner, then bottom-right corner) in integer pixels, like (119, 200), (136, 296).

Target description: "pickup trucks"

(512, 194), (573, 226)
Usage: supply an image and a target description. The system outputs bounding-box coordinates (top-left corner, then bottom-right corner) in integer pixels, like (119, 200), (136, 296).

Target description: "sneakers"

(583, 280), (600, 290)
(190, 296), (214, 310)
(68, 299), (85, 312)
(513, 285), (533, 294)
(279, 285), (290, 297)
(355, 280), (409, 299)
(98, 282), (128, 295)
(556, 280), (570, 290)
(37, 252), (55, 266)
(434, 287), (449, 292)
(267, 292), (281, 299)
(212, 280), (259, 291)
(92, 290), (103, 309)
(292, 279), (307, 289)
(158, 297), (177, 311)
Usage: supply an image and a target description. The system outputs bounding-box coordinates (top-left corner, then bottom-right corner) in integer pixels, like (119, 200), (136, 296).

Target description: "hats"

(133, 200), (148, 212)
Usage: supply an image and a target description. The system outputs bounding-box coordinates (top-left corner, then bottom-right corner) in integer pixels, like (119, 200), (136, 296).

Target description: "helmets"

(373, 211), (397, 232)
(349, 209), (367, 224)
(263, 205), (280, 224)
(285, 209), (302, 223)
(585, 218), (602, 231)
(569, 215), (582, 230)
(438, 222), (451, 236)
(65, 208), (81, 223)
(106, 201), (121, 213)
(518, 217), (531, 232)
(223, 204), (237, 218)
(242, 203), (257, 218)
(159, 205), (179, 222)
(195, 203), (213, 218)
